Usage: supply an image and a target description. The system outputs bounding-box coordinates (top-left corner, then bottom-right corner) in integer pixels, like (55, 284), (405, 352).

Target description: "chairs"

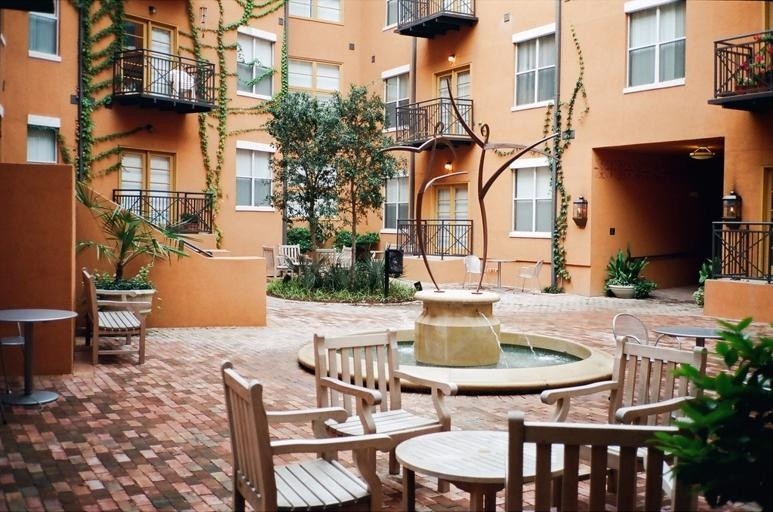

(506, 410), (696, 510)
(461, 254), (487, 293)
(274, 242), (352, 277)
(170, 68), (196, 102)
(220, 359), (394, 510)
(613, 313), (683, 350)
(513, 260), (543, 295)
(312, 327), (459, 492)
(543, 337), (708, 491)
(80, 266), (146, 364)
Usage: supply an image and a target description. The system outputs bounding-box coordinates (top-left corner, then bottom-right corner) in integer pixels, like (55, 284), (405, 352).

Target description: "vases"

(735, 84), (766, 93)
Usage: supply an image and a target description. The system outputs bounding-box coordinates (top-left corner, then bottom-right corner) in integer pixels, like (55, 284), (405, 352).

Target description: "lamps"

(572, 194), (588, 231)
(689, 145), (717, 162)
(722, 189), (744, 232)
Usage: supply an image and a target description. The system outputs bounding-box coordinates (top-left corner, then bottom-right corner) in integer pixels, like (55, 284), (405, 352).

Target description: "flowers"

(735, 34), (772, 86)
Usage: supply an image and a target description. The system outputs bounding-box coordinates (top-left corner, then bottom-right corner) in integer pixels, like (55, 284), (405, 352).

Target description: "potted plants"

(74, 179), (193, 321)
(181, 212), (199, 235)
(604, 248), (658, 297)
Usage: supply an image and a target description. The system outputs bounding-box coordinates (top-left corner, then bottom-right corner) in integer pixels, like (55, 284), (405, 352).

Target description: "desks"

(651, 326), (747, 390)
(486, 259), (513, 295)
(1, 306), (80, 408)
(395, 429), (565, 511)
